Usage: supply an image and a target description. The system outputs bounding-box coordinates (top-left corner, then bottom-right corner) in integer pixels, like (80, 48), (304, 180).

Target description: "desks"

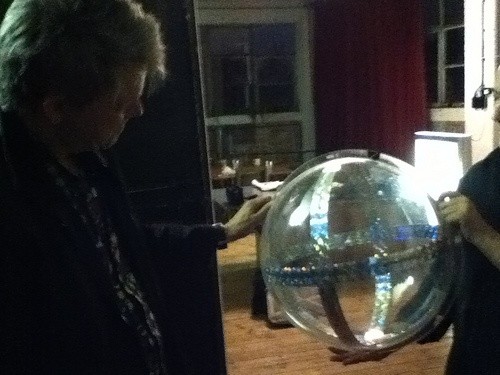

(209, 156), (294, 318)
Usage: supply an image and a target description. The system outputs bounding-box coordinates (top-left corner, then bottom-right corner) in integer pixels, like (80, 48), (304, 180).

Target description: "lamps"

(470, 85), (499, 114)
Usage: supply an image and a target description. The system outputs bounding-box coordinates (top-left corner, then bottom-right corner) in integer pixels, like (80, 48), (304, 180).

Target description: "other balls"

(255, 146), (465, 355)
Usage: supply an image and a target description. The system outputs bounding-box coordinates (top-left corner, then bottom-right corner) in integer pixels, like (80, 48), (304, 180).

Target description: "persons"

(328, 52), (500, 375)
(1, 0), (275, 374)
